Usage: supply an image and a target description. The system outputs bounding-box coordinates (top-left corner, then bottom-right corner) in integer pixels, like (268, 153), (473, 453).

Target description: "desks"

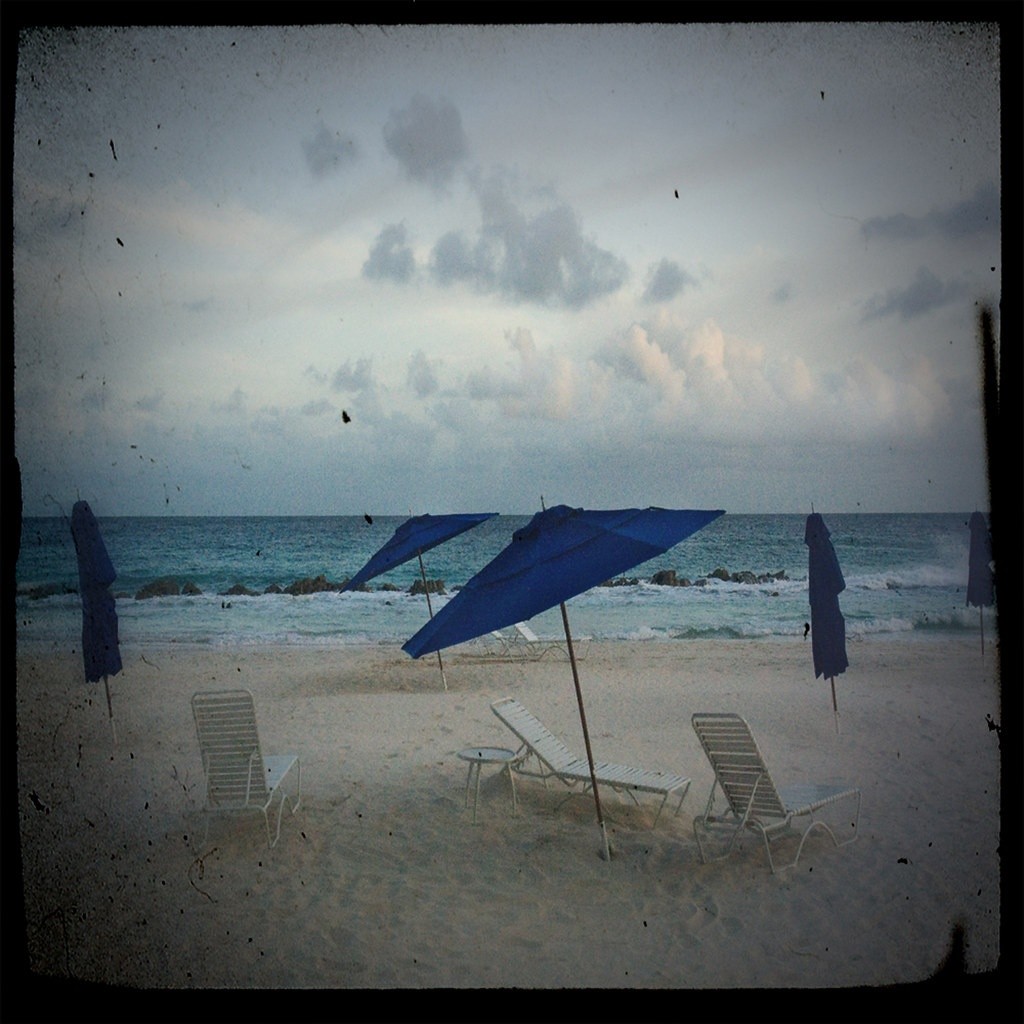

(457, 745), (518, 826)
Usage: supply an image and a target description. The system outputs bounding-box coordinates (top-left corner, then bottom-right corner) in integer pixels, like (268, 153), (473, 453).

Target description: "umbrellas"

(339, 509), (500, 693)
(965, 506), (994, 659)
(71, 489), (125, 745)
(401, 495), (727, 867)
(804, 502), (849, 712)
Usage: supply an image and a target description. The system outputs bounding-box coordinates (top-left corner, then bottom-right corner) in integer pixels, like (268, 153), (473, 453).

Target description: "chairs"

(190, 687), (302, 855)
(484, 621), (594, 663)
(491, 696), (691, 829)
(691, 712), (864, 874)
